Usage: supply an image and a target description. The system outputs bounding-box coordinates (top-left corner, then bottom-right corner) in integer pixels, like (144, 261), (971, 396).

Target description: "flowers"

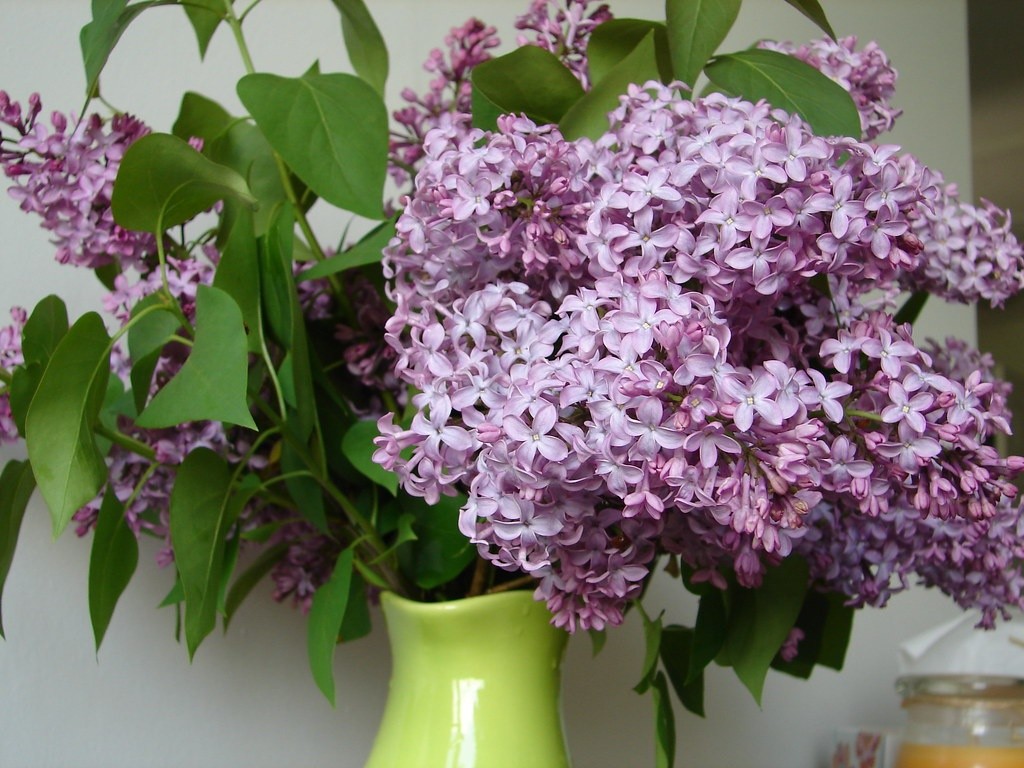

(0, 1), (1024, 660)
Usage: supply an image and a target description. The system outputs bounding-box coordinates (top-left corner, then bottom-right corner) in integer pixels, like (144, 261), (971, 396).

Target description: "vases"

(365, 578), (575, 768)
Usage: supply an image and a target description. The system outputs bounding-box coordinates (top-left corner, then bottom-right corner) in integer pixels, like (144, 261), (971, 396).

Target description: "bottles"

(891, 674), (1024, 768)
